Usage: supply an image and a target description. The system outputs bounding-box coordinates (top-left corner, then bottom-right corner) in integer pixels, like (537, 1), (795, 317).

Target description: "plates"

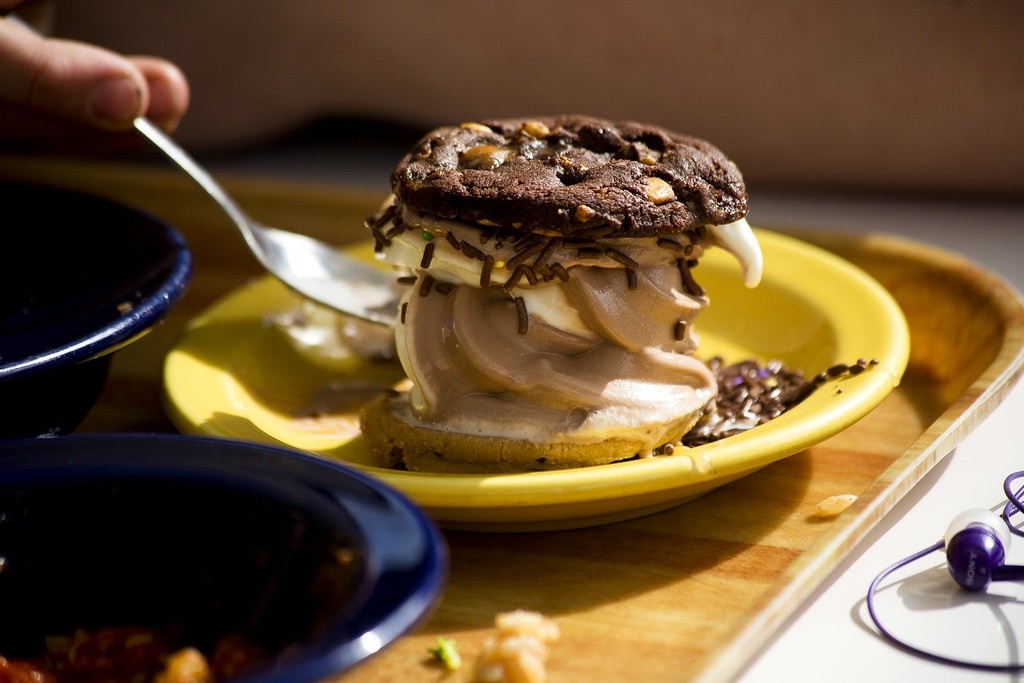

(167, 228), (911, 537)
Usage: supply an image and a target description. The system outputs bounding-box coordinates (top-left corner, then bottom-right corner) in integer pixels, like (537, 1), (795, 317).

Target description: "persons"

(0, 0), (192, 146)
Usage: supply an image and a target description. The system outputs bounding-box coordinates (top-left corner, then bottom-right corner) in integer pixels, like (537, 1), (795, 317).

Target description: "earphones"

(946, 507), (1011, 593)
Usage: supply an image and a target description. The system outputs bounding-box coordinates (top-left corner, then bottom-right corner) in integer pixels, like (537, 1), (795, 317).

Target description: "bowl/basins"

(0, 435), (439, 683)
(0, 178), (191, 433)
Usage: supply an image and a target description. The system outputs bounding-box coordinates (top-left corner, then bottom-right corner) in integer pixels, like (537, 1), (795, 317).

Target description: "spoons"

(134, 117), (404, 328)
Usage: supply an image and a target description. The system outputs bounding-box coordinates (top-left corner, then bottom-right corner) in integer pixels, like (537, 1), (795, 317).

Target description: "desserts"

(358, 113), (814, 468)
